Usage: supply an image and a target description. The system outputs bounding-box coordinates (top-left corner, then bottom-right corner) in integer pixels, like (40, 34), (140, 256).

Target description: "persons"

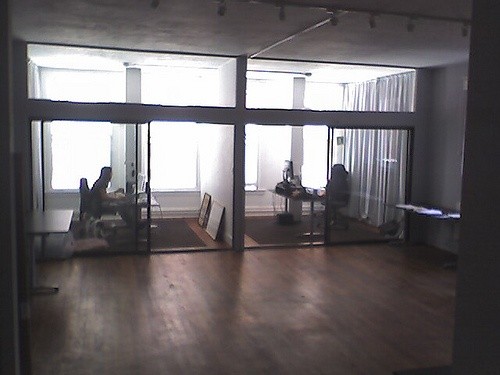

(90, 167), (152, 231)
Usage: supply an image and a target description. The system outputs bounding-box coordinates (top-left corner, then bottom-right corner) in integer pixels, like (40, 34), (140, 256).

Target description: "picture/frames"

(198, 193), (211, 228)
(205, 200), (225, 241)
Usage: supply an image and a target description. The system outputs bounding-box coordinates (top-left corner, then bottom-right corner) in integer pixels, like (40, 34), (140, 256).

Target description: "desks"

(105, 192), (162, 229)
(269, 187), (323, 240)
(23, 209), (75, 290)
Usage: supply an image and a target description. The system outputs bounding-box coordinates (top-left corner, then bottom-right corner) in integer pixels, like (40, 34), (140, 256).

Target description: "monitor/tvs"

(283, 160), (294, 182)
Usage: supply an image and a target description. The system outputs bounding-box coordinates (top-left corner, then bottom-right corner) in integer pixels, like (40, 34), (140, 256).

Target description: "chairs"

(79, 178), (105, 226)
(321, 200), (349, 231)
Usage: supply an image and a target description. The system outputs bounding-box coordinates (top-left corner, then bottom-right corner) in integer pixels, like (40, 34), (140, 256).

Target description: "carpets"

(70, 221), (207, 249)
(244, 215), (384, 244)
